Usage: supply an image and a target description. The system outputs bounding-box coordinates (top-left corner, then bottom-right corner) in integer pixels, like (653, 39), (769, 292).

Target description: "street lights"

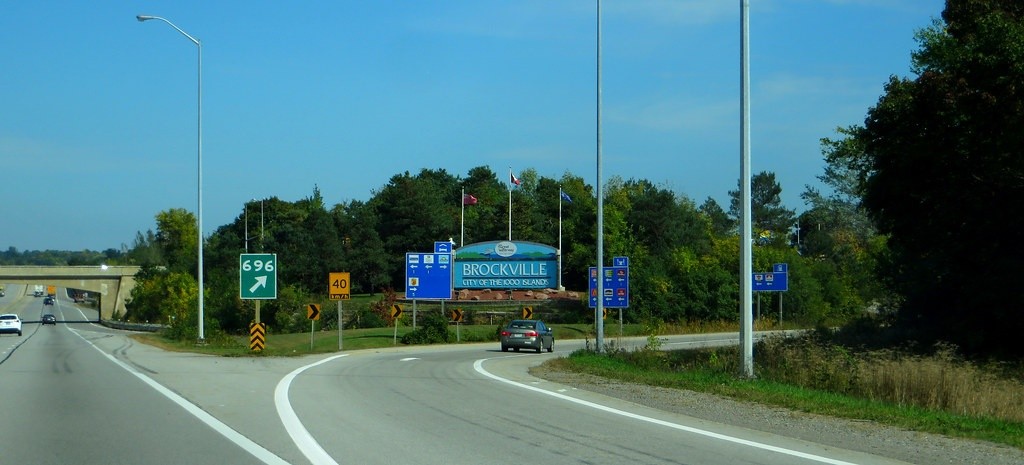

(136, 14), (205, 346)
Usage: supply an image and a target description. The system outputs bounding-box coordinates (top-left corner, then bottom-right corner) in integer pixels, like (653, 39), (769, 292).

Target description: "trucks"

(48, 285), (57, 298)
(35, 284), (44, 295)
(75, 293), (85, 303)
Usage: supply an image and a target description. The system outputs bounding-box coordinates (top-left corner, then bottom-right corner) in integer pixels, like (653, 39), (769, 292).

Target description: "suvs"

(0, 289), (6, 297)
(0, 313), (24, 336)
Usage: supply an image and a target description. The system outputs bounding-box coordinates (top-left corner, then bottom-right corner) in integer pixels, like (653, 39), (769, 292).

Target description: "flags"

(511, 173), (519, 185)
(464, 194), (477, 204)
(561, 191), (571, 203)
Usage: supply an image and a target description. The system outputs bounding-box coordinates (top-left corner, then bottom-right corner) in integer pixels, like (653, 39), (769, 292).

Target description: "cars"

(34, 291), (42, 297)
(42, 314), (57, 326)
(43, 297), (55, 306)
(501, 319), (555, 354)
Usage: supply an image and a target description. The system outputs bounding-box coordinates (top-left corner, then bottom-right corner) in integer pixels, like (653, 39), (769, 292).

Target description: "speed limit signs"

(329, 272), (351, 301)
